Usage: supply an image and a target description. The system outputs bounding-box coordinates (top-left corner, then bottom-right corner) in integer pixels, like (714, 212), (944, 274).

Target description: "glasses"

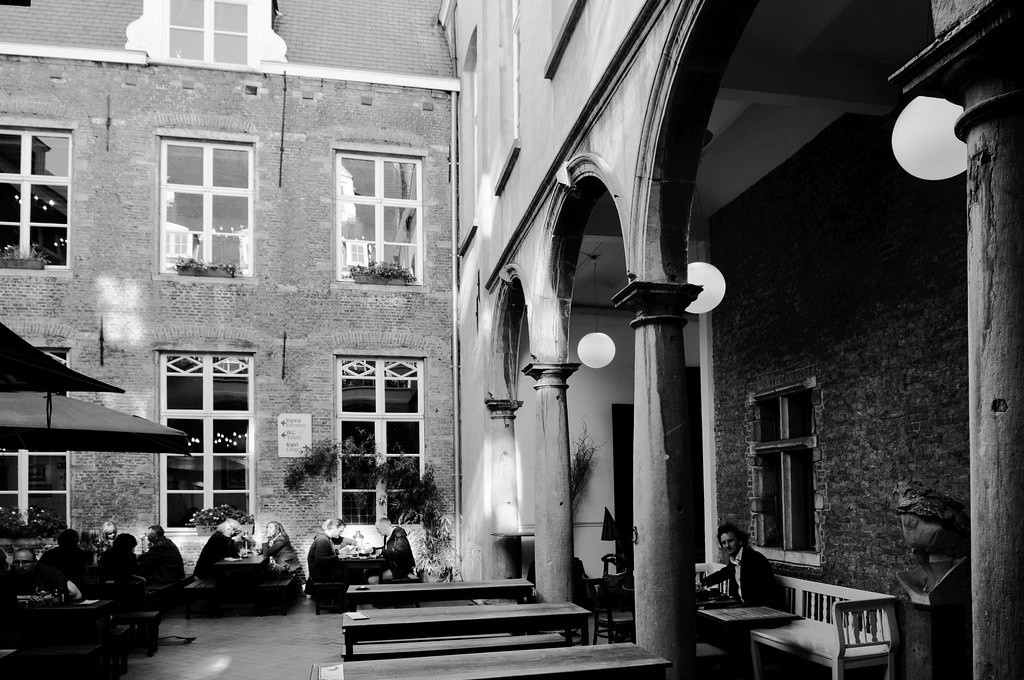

(13, 558), (35, 566)
(2, 562), (11, 571)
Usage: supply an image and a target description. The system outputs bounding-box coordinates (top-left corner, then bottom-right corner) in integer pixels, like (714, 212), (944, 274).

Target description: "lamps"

(239, 531), (252, 550)
(141, 532), (150, 553)
(892, 96), (967, 180)
(353, 531), (365, 547)
(576, 252), (616, 368)
(683, 129), (726, 314)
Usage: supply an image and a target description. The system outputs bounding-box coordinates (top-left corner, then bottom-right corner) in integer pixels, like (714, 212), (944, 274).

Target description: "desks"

(695, 590), (738, 608)
(342, 601), (593, 662)
(491, 531), (535, 605)
(696, 606), (805, 680)
(346, 578), (535, 612)
(213, 550), (269, 616)
(338, 554), (386, 612)
(309, 642), (673, 680)
(7, 599), (120, 680)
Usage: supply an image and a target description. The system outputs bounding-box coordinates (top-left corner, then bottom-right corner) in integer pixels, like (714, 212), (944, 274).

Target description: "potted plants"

(348, 261), (417, 285)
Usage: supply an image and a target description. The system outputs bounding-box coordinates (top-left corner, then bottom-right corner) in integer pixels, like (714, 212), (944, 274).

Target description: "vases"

(196, 524), (254, 536)
(177, 266), (234, 277)
(0, 257), (44, 270)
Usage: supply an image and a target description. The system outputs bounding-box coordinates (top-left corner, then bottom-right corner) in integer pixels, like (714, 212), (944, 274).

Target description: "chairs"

(594, 553), (633, 644)
(582, 572), (636, 645)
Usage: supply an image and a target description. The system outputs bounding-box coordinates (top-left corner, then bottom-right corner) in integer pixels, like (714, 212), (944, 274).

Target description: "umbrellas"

(0, 323), (190, 453)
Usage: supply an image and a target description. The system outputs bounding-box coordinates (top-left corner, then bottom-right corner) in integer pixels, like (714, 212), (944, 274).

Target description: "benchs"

(695, 563), (901, 680)
(183, 579), (225, 621)
(7, 640), (103, 680)
(312, 581), (346, 616)
(340, 632), (575, 662)
(114, 610), (162, 657)
(386, 573), (421, 608)
(143, 573), (192, 614)
(254, 573), (298, 617)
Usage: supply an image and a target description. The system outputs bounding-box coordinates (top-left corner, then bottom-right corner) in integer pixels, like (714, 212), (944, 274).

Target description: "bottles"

(60, 578), (69, 604)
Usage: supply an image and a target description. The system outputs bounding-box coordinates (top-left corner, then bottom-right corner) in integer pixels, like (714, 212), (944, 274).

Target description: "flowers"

(184, 504), (255, 532)
(0, 244), (51, 264)
(0, 505), (66, 545)
(175, 255), (236, 274)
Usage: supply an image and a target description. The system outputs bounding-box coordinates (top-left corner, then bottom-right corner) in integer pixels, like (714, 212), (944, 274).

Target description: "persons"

(696, 523), (775, 606)
(365, 518), (416, 584)
(0, 548), (82, 603)
(138, 525), (186, 581)
(193, 519), (306, 585)
(78, 521), (137, 576)
(307, 518), (356, 582)
(40, 529), (86, 587)
